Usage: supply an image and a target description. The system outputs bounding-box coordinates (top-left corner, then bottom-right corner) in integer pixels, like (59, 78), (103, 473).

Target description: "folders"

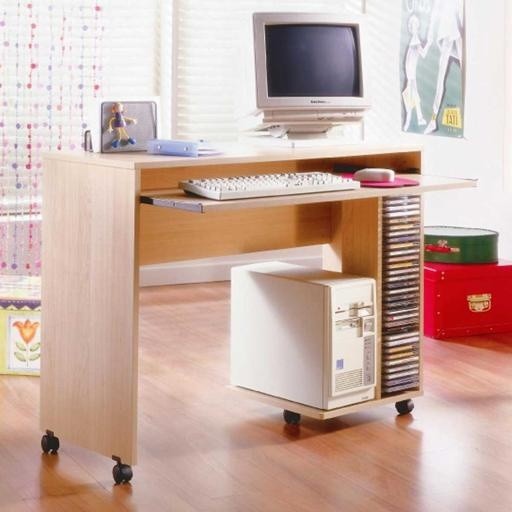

(147, 140), (223, 158)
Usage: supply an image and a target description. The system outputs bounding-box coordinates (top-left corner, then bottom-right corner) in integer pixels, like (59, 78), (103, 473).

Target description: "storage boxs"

(422, 263), (512, 341)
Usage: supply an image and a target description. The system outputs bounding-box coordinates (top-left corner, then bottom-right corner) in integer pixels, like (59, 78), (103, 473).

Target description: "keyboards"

(177, 171), (360, 201)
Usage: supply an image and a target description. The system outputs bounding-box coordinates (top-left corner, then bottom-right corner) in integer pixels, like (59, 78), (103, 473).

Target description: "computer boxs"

(231, 261), (377, 411)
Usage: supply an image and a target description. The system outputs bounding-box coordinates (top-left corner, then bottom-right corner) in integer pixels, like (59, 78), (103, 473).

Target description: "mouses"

(353, 168), (395, 182)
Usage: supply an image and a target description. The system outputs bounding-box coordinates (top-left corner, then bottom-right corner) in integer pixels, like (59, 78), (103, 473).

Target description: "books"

(381, 196), (422, 397)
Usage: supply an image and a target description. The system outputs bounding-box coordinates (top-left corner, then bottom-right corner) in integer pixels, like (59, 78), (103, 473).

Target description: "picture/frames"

(2, 305), (42, 379)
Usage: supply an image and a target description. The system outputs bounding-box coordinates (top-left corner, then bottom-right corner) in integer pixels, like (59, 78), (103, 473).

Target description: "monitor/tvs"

(252, 12), (374, 139)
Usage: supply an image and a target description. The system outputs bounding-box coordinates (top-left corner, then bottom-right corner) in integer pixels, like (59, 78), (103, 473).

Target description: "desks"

(32, 137), (426, 488)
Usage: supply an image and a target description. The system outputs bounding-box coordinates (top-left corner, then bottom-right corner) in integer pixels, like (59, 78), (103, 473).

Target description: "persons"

(420, 0), (464, 137)
(106, 102), (138, 149)
(397, 14), (433, 133)
